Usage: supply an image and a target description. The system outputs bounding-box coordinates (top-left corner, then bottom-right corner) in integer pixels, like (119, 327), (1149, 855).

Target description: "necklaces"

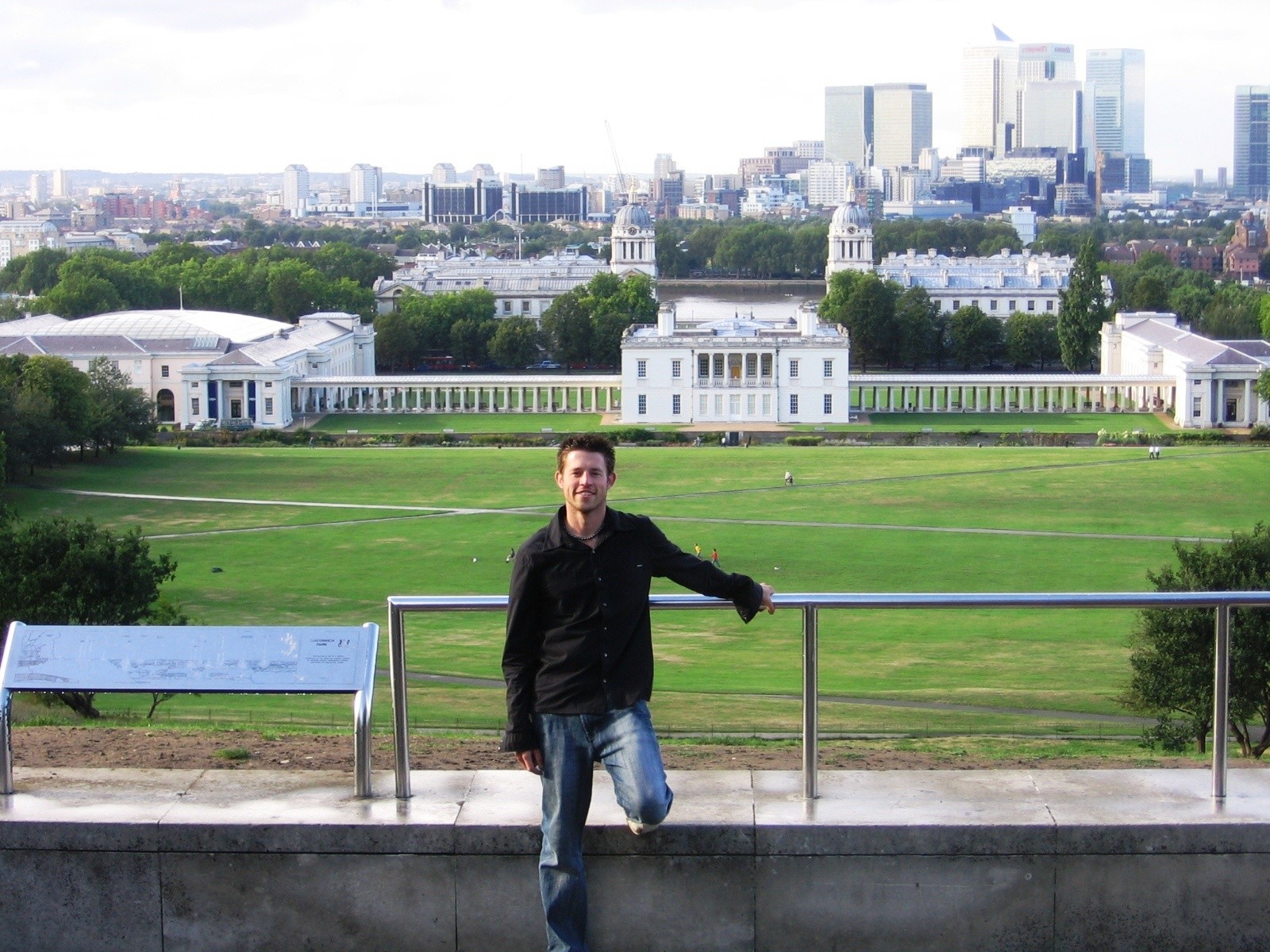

(563, 512), (606, 541)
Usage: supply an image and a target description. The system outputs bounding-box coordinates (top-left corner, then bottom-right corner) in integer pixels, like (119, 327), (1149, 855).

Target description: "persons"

(1148, 445), (1161, 460)
(502, 433), (774, 952)
(785, 470), (793, 484)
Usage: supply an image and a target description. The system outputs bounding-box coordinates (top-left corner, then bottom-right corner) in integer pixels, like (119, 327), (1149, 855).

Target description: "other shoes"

(628, 819), (661, 835)
